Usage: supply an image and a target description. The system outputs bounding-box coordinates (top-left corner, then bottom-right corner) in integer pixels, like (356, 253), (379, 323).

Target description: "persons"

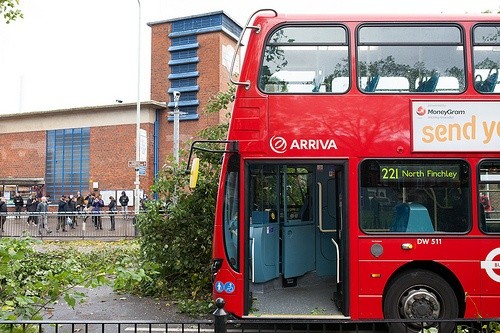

(371, 196), (382, 228)
(480, 192), (490, 218)
(0, 191), (151, 234)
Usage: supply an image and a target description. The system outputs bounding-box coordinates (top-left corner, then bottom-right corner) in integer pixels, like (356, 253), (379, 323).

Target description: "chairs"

(298, 194), (310, 221)
(311, 66), (500, 93)
(391, 203), (410, 233)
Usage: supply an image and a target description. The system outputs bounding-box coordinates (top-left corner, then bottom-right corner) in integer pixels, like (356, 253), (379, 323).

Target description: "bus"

(184, 7), (500, 333)
